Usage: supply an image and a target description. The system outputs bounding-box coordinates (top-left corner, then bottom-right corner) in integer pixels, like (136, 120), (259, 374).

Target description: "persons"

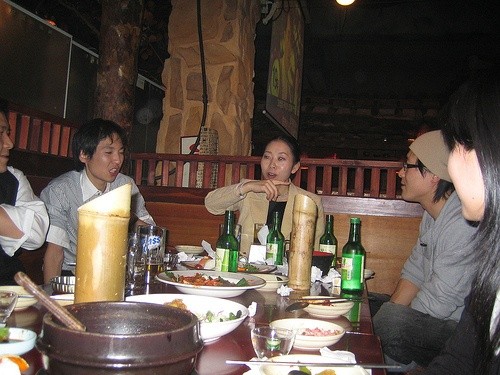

(0, 97), (49, 285)
(41, 118), (157, 288)
(426, 77), (500, 375)
(205, 133), (326, 258)
(367, 129), (480, 375)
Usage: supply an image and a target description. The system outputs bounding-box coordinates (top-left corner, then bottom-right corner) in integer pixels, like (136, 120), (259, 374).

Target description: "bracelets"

(240, 183), (245, 195)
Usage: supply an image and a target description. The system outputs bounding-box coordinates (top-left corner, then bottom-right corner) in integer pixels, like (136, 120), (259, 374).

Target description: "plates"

(261, 354), (369, 375)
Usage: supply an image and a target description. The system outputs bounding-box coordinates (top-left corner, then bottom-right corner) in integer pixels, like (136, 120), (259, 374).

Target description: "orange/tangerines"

(1, 355), (30, 373)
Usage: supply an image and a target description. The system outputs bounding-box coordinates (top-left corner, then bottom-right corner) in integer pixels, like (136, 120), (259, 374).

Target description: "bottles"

(319, 213), (339, 269)
(340, 217), (367, 293)
(338, 292), (362, 352)
(212, 208), (240, 272)
(265, 210), (284, 265)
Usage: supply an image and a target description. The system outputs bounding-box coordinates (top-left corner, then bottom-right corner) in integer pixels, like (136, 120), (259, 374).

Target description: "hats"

(409, 130), (452, 182)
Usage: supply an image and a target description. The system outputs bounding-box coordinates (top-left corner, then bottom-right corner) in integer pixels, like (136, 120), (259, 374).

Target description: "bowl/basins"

(284, 249), (335, 279)
(175, 244), (205, 256)
(41, 295), (204, 375)
(0, 256), (376, 375)
(192, 334), (245, 375)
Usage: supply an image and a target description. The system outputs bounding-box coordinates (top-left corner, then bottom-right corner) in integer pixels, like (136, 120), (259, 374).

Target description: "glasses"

(402, 161), (423, 171)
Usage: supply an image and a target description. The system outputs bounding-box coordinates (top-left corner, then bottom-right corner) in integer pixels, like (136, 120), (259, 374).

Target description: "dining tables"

(0, 252), (388, 375)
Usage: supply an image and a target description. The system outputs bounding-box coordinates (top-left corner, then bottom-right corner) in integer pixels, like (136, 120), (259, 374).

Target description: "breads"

(199, 256), (215, 269)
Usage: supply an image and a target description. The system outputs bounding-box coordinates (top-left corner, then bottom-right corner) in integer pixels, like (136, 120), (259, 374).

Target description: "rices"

(194, 310), (225, 321)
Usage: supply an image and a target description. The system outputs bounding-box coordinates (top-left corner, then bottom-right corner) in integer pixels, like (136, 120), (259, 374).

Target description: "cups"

(123, 225), (169, 290)
(252, 223), (268, 246)
(217, 223), (242, 246)
(250, 325), (296, 361)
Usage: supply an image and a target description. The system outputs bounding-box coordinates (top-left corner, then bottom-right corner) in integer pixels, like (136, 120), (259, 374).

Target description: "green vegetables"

(164, 263), (258, 286)
(206, 310), (242, 321)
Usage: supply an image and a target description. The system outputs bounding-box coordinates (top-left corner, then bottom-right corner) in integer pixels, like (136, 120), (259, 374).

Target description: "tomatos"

(195, 264), (202, 269)
